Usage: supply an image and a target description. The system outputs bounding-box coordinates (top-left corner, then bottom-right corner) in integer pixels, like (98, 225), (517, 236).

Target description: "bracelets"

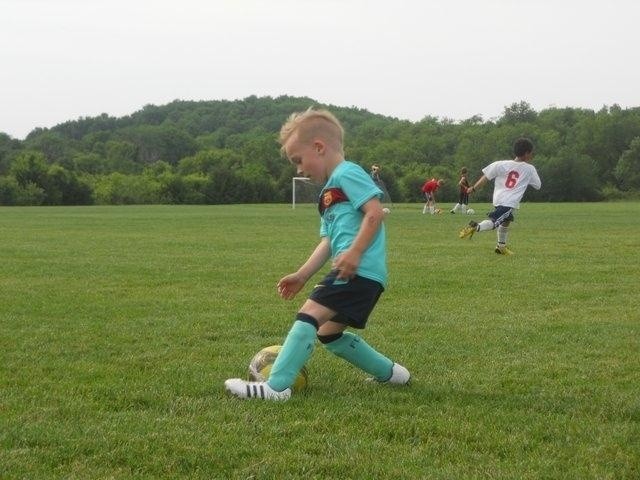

(472, 185), (476, 193)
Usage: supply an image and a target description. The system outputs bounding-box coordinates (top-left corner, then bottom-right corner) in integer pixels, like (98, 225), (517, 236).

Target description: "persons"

(420, 178), (446, 216)
(459, 138), (542, 257)
(221, 105), (411, 404)
(370, 162), (381, 182)
(449, 166), (470, 215)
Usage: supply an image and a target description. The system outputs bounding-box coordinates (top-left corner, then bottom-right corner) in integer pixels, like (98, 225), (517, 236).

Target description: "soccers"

(467, 209), (475, 215)
(248, 345), (309, 392)
(382, 207), (391, 216)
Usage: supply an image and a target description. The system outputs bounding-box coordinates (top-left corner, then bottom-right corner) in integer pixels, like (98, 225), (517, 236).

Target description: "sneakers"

(366, 362), (411, 385)
(449, 209), (455, 214)
(225, 378), (292, 400)
(459, 220), (477, 239)
(496, 245), (511, 255)
